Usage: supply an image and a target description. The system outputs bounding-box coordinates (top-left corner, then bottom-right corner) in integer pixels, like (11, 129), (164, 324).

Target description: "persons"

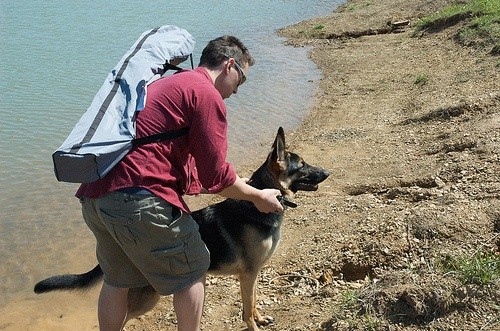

(75, 35), (284, 331)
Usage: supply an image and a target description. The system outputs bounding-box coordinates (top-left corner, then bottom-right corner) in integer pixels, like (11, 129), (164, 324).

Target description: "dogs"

(33, 125), (331, 331)
